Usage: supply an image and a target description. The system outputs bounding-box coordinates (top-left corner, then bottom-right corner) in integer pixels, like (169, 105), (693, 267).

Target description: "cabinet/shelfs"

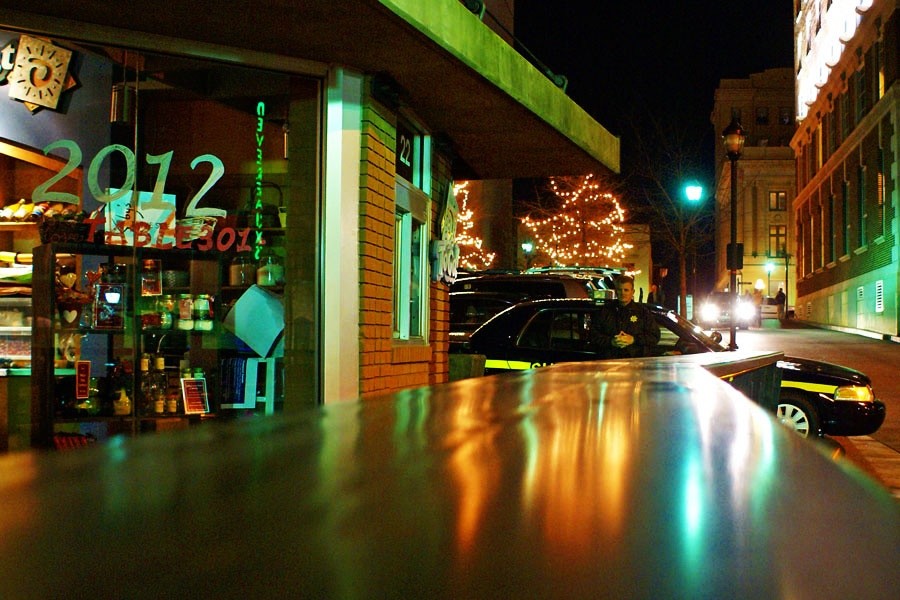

(0, 239), (267, 449)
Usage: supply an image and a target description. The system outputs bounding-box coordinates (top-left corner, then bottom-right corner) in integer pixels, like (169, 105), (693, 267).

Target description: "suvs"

(449, 264), (635, 333)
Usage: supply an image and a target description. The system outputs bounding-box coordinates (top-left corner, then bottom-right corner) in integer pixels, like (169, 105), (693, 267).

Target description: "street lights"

(764, 261), (774, 296)
(722, 110), (750, 350)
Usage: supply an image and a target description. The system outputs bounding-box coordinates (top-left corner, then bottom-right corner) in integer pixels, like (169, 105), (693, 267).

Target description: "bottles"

(228, 254), (257, 286)
(160, 293), (213, 331)
(0, 198), (107, 222)
(257, 256), (284, 286)
(141, 352), (204, 414)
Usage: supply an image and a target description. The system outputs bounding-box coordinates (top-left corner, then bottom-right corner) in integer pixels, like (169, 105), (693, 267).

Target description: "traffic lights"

(684, 176), (700, 211)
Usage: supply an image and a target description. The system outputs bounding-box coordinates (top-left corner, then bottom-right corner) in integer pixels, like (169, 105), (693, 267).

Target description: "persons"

(647, 285), (661, 305)
(588, 276), (660, 360)
(775, 288), (786, 321)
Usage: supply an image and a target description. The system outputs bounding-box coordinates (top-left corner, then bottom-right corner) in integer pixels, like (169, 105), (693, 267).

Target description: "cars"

(697, 291), (795, 331)
(448, 285), (887, 443)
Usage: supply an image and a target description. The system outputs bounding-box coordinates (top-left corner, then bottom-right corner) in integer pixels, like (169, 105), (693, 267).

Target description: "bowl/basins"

(162, 270), (189, 287)
(12, 360), (31, 367)
(54, 360), (67, 368)
